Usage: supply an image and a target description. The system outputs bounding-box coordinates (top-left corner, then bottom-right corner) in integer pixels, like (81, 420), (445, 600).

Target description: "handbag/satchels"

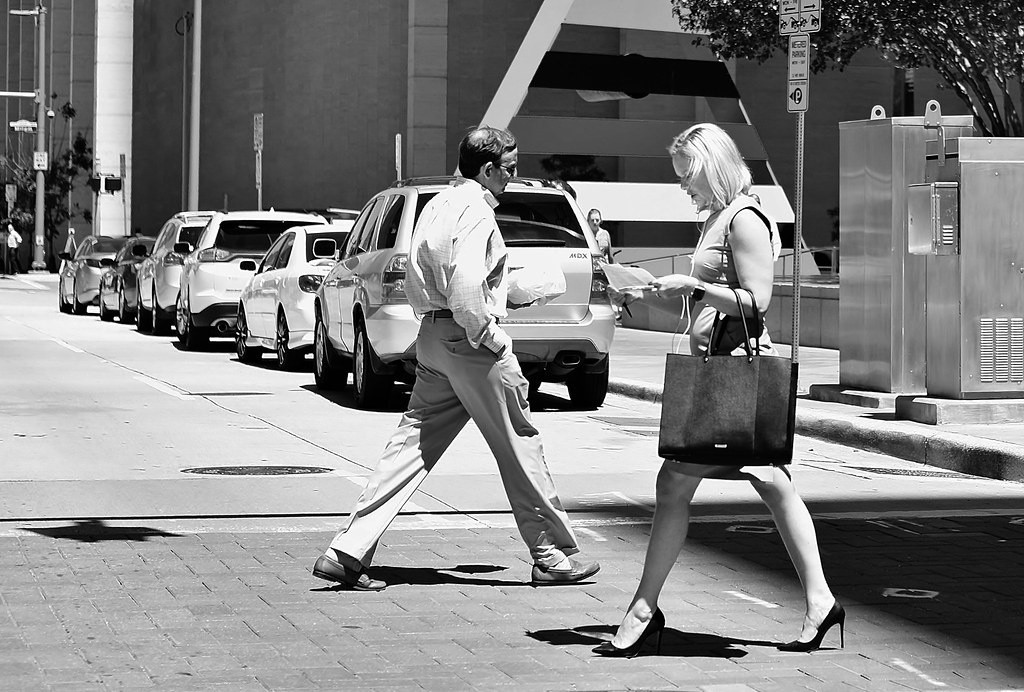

(659, 289), (798, 466)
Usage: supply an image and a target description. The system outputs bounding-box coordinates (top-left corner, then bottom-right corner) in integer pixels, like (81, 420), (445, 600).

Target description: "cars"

(98, 236), (157, 324)
(132, 211), (221, 335)
(235, 224), (356, 370)
(58, 235), (129, 314)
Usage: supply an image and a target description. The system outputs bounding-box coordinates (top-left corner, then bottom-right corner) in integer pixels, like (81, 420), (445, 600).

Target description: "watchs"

(692, 278), (707, 303)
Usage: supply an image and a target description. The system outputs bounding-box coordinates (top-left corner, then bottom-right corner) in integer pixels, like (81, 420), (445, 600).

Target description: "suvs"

(314, 176), (616, 407)
(174, 209), (329, 349)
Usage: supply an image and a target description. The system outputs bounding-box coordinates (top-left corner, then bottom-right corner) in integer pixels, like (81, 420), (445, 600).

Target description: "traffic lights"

(92, 178), (100, 191)
(105, 177), (122, 191)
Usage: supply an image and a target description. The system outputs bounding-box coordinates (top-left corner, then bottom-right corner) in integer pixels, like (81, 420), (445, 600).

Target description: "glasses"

(494, 163), (516, 174)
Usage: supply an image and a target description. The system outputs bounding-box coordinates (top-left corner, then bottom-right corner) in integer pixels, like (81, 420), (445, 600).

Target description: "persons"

(313, 130), (600, 592)
(587, 209), (614, 264)
(590, 121), (846, 657)
(6, 224), (25, 275)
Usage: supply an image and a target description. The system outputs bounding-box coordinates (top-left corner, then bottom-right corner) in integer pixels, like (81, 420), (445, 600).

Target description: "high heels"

(778, 600), (846, 651)
(592, 607), (665, 657)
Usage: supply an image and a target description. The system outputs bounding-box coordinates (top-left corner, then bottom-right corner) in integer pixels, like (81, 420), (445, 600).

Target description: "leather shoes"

(312, 555), (386, 591)
(531, 557), (599, 584)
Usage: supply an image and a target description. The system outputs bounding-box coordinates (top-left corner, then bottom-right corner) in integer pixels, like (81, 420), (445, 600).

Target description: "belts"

(425, 310), (453, 319)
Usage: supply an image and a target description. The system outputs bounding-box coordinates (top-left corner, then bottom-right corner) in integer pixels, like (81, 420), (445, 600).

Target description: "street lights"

(9, 5), (51, 275)
